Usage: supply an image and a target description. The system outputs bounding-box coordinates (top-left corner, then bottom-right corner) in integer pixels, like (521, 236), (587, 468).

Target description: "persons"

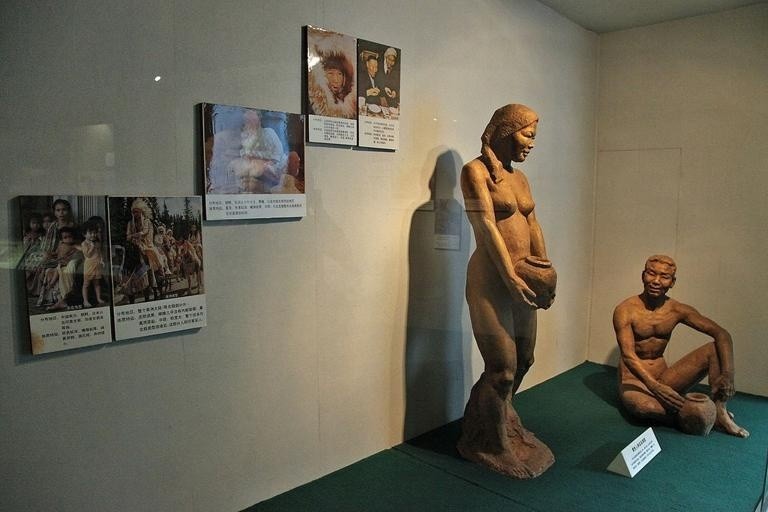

(226, 109), (288, 196)
(19, 197), (209, 312)
(456, 99), (555, 482)
(376, 47), (401, 111)
(607, 251), (753, 441)
(360, 55), (389, 108)
(308, 53), (352, 118)
